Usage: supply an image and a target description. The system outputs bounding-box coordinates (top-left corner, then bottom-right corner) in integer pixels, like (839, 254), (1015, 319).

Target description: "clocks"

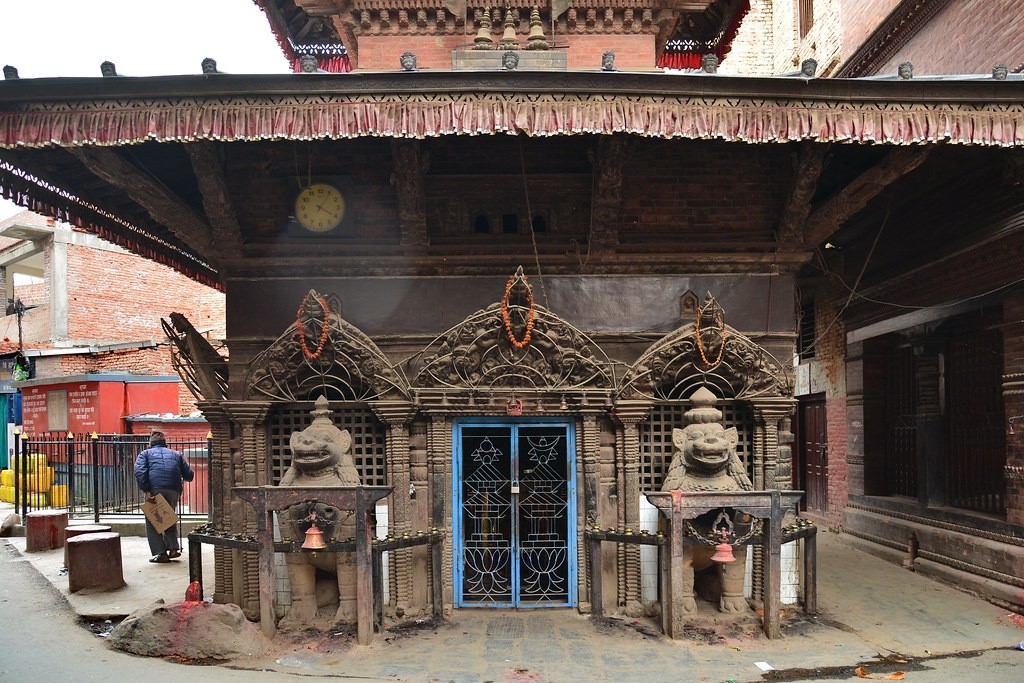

(295, 183), (345, 233)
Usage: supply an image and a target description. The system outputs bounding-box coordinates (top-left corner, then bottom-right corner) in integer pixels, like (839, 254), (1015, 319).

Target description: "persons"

(992, 63), (1007, 80)
(133, 431), (194, 563)
(898, 61), (914, 80)
(602, 50), (615, 71)
(300, 54), (318, 73)
(3, 65), (19, 80)
(101, 61), (116, 77)
(502, 51), (520, 70)
(202, 57), (216, 74)
(702, 53), (718, 74)
(799, 58), (818, 78)
(400, 51), (416, 71)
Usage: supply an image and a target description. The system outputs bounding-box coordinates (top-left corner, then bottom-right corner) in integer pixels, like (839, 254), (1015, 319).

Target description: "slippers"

(169, 553), (181, 557)
(149, 556), (171, 563)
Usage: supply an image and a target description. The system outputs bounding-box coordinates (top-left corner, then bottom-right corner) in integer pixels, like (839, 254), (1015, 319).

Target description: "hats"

(150, 431), (165, 441)
